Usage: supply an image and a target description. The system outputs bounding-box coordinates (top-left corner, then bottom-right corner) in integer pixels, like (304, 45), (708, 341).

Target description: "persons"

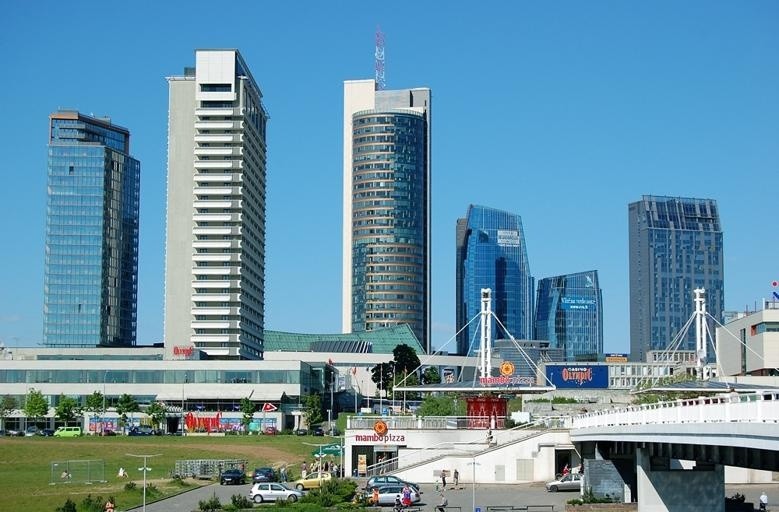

(300, 458), (340, 480)
(353, 484), (412, 512)
(453, 469), (460, 489)
(434, 480), (439, 492)
(437, 493), (448, 512)
(440, 469), (447, 489)
(61, 469), (70, 482)
(759, 491), (768, 512)
(574, 463), (581, 471)
(106, 496), (115, 512)
(279, 464), (288, 483)
(483, 426), (493, 448)
(378, 455), (387, 474)
(116, 467), (130, 479)
(378, 456), (381, 466)
(563, 462), (569, 477)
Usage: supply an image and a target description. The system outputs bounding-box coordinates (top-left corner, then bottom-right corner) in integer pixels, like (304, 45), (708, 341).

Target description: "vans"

(53, 426), (82, 438)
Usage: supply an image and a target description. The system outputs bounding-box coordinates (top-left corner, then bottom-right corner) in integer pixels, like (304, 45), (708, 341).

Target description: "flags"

(352, 366), (357, 376)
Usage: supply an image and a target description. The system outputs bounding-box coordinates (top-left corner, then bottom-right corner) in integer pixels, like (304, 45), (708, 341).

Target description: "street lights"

(104, 370), (109, 420)
(181, 374), (187, 433)
(125, 454), (163, 512)
(330, 371), (335, 421)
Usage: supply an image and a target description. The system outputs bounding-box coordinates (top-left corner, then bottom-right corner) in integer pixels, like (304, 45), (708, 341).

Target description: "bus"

(360, 398), (425, 415)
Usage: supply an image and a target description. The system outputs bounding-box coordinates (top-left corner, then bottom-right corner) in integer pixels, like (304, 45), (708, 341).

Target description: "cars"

(326, 428), (340, 435)
(249, 482), (305, 502)
(294, 472), (332, 490)
(1, 428), (55, 436)
(221, 470), (245, 485)
(313, 429), (324, 436)
(129, 426), (161, 435)
(266, 427), (275, 434)
(297, 429), (307, 435)
(360, 475), (420, 505)
(546, 473), (584, 492)
(100, 430), (116, 435)
(253, 468), (277, 484)
